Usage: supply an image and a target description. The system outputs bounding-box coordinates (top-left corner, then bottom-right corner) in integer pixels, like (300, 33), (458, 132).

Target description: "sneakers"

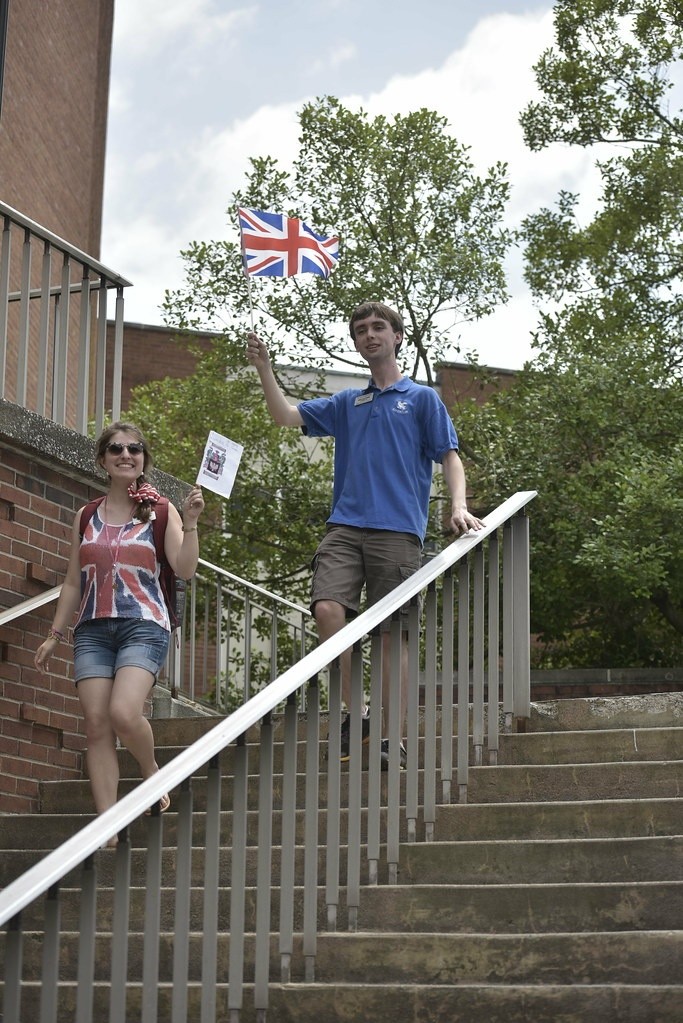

(380, 739), (406, 770)
(325, 706), (371, 761)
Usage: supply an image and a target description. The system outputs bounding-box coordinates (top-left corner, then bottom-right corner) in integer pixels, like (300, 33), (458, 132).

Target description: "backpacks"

(80, 496), (178, 632)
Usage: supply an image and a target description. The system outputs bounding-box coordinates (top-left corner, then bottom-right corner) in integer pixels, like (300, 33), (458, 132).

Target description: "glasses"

(104, 443), (145, 455)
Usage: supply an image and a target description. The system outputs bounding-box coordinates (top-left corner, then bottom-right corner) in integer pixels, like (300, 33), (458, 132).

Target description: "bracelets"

(46, 628), (64, 643)
(181, 526), (197, 533)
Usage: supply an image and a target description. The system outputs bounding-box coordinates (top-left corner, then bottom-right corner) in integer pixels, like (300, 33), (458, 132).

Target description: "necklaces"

(104, 492), (137, 590)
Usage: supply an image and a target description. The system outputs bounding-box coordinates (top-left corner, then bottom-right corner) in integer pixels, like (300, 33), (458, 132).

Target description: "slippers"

(146, 794), (171, 816)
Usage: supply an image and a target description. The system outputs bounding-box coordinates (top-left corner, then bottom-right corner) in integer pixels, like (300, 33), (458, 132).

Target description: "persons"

(33, 420), (206, 847)
(247, 302), (488, 766)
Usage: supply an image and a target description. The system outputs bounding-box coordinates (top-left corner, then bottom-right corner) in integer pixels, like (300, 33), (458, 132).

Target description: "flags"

(237, 206), (342, 281)
